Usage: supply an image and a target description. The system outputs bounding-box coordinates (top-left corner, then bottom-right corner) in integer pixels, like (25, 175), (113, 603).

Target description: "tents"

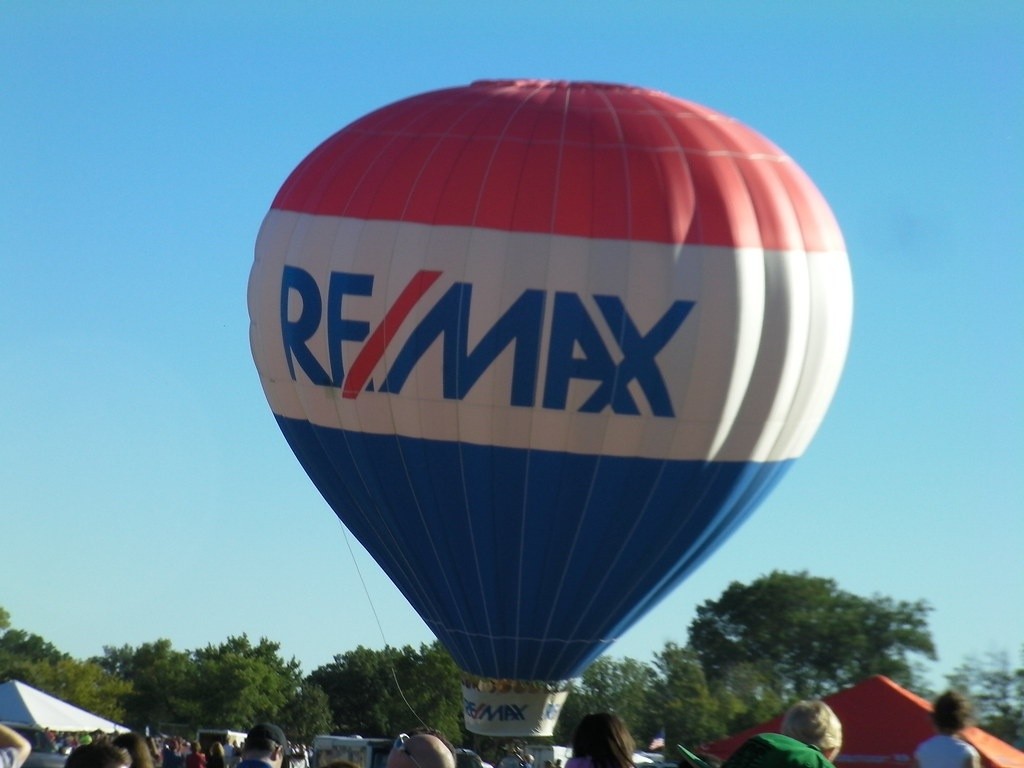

(699, 673), (1024, 768)
(0, 679), (131, 733)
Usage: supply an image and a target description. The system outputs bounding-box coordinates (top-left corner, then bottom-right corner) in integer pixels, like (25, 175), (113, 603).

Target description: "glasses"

(395, 732), (421, 768)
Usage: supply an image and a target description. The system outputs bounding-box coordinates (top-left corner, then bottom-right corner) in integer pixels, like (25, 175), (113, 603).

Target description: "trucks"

(312, 734), (395, 768)
(196, 729), (248, 755)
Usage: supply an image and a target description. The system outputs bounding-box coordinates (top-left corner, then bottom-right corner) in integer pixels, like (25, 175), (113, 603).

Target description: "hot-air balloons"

(243, 80), (856, 768)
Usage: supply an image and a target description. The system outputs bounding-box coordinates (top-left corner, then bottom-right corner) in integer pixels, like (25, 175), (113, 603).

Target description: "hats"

(89, 730), (101, 735)
(677, 733), (836, 768)
(245, 724), (292, 756)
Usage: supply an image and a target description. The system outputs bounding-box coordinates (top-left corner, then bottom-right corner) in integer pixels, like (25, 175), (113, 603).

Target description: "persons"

(0, 723), (313, 768)
(781, 699), (842, 762)
(385, 726), (562, 768)
(913, 690), (981, 768)
(563, 710), (656, 768)
